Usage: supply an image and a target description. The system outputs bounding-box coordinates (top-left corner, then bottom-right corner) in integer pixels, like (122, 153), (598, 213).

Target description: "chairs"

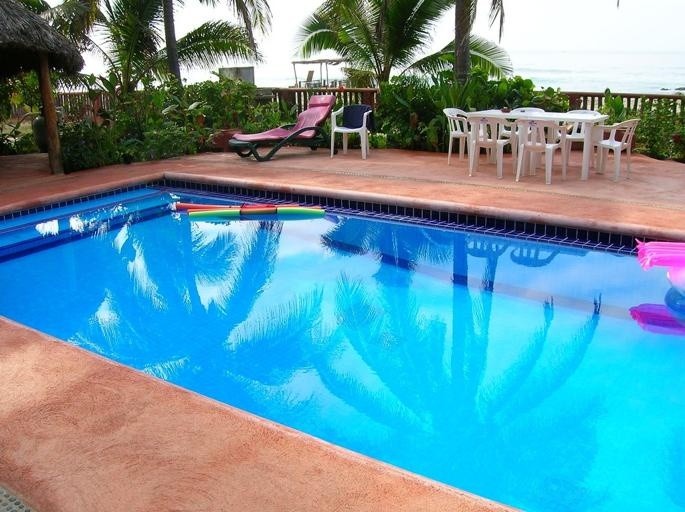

(227, 94), (337, 163)
(441, 105), (640, 185)
(329, 104), (374, 161)
